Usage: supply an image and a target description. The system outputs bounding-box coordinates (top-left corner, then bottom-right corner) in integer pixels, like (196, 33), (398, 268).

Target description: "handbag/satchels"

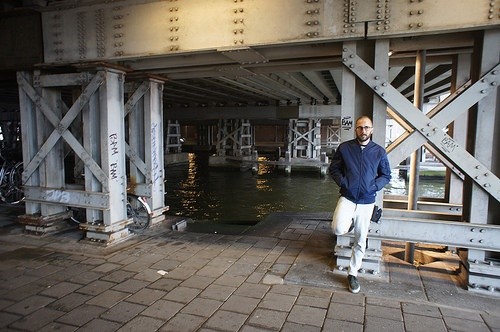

(371, 205), (383, 223)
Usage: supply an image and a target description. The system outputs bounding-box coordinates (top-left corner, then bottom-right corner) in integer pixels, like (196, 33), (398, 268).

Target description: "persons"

(329, 115), (392, 293)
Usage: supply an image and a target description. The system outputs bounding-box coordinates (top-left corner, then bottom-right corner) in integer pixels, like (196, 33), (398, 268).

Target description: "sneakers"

(348, 218), (355, 232)
(347, 274), (361, 294)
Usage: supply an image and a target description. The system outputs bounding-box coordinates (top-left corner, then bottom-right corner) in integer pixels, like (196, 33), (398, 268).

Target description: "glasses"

(356, 126), (373, 131)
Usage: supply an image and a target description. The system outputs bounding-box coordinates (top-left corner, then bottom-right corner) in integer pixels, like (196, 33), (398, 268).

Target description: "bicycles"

(0, 150), (26, 206)
(67, 173), (154, 234)
